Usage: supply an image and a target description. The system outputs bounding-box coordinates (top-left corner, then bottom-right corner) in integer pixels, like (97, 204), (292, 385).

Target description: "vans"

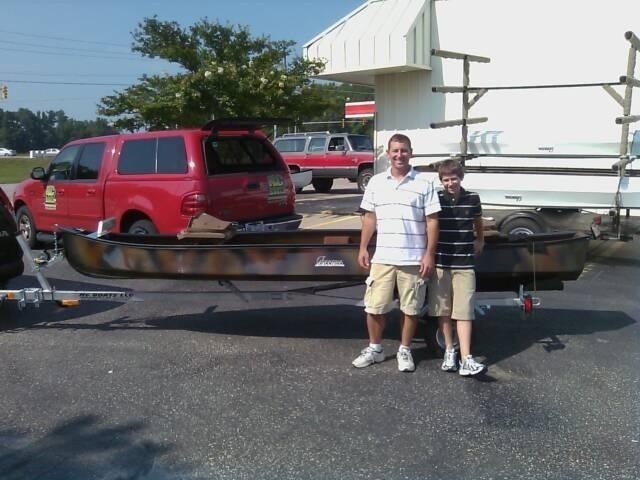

(13, 116), (303, 249)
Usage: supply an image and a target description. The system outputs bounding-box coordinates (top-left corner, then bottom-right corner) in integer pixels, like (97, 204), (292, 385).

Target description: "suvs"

(271, 131), (375, 193)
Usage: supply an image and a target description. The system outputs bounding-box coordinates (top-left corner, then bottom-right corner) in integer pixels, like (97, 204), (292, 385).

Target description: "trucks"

(304, 0), (640, 242)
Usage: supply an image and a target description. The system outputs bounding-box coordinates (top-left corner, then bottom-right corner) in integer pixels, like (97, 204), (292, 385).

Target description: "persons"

(420, 156), (489, 381)
(349, 133), (445, 376)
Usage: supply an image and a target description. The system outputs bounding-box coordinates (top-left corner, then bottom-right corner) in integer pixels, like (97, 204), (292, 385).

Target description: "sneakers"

(396, 347), (415, 372)
(441, 350), (457, 372)
(351, 347), (385, 368)
(458, 355), (488, 376)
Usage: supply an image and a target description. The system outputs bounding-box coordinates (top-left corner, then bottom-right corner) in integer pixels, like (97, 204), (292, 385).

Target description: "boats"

(55, 216), (590, 290)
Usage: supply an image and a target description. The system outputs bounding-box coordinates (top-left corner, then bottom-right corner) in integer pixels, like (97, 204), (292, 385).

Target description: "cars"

(0, 148), (16, 157)
(44, 148), (59, 157)
